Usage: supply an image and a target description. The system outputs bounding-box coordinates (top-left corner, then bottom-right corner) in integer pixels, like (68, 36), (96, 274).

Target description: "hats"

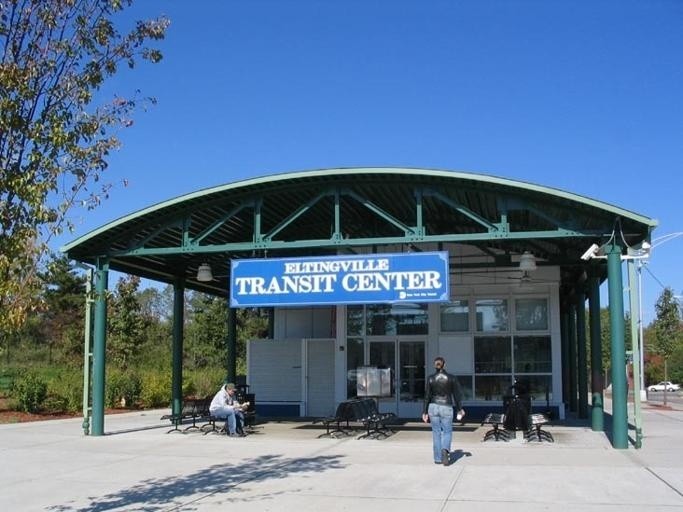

(226, 382), (238, 392)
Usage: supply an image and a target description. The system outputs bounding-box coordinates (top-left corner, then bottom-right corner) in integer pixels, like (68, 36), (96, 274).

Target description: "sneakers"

(228, 429), (247, 437)
(440, 448), (452, 467)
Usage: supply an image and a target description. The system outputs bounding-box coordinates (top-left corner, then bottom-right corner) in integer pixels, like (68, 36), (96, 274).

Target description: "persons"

(209, 382), (248, 438)
(422, 357), (465, 466)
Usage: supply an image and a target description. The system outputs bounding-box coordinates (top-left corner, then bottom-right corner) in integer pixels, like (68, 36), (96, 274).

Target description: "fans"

(505, 270), (545, 287)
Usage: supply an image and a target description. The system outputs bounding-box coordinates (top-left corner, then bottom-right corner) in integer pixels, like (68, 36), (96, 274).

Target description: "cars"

(347, 368), (361, 382)
(647, 380), (680, 393)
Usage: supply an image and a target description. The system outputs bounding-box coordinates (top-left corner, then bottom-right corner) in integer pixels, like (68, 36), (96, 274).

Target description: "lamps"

(196, 262), (213, 282)
(517, 250), (538, 271)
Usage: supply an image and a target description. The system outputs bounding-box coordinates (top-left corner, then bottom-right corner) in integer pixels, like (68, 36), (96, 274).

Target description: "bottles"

(456, 411), (463, 422)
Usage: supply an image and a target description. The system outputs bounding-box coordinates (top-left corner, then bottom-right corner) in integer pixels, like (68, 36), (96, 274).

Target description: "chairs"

(479, 392), (557, 445)
(163, 390), (265, 436)
(309, 395), (399, 439)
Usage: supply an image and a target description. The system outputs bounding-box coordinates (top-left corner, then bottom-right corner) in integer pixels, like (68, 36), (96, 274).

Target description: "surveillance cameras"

(580, 242), (599, 262)
